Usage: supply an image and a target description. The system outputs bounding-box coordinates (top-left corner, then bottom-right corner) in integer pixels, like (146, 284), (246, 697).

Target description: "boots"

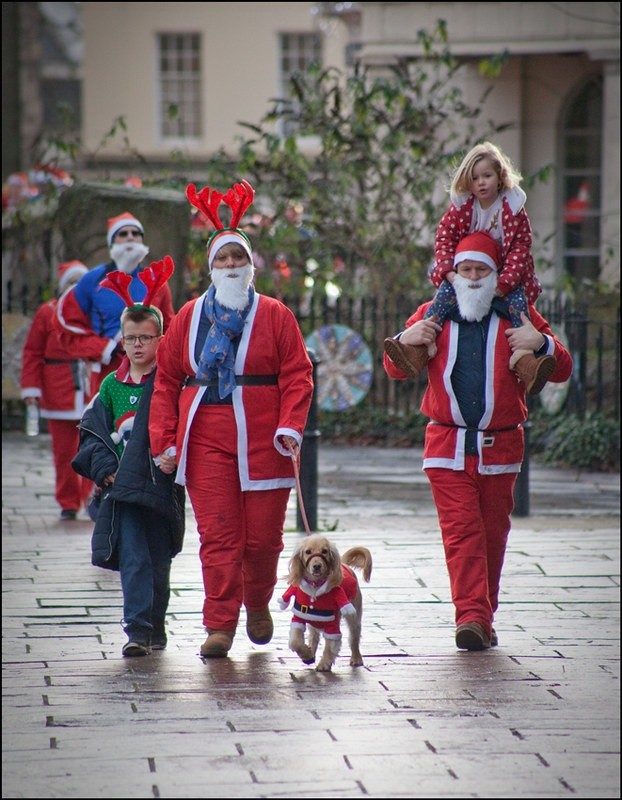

(509, 348), (557, 396)
(383, 335), (437, 379)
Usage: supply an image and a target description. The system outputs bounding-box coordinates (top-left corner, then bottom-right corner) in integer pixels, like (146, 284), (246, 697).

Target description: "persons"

(148, 179), (314, 658)
(19, 261), (95, 521)
(71, 255), (186, 657)
(52, 211), (176, 399)
(383, 230), (573, 650)
(384, 142), (557, 395)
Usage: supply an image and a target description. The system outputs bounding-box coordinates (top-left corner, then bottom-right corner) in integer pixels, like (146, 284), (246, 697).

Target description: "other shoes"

(121, 641), (148, 656)
(61, 511), (75, 518)
(200, 629), (234, 656)
(88, 494), (101, 519)
(491, 627), (498, 644)
(455, 622), (491, 650)
(246, 609), (274, 644)
(150, 634), (167, 649)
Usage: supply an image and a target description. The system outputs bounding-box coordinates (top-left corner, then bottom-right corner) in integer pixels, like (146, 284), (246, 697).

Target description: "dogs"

(288, 533), (371, 671)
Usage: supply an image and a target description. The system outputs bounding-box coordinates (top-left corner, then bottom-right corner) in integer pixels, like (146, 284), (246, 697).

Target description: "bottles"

(25, 398), (39, 437)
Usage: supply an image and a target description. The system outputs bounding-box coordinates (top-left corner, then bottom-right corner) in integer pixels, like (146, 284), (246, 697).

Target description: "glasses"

(122, 334), (157, 344)
(119, 231), (143, 237)
(71, 280), (78, 285)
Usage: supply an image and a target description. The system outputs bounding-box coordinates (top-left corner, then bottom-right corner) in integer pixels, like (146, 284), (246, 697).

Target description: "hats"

(106, 212), (145, 246)
(204, 226), (254, 268)
(121, 301), (165, 335)
(453, 231), (502, 271)
(57, 259), (89, 294)
